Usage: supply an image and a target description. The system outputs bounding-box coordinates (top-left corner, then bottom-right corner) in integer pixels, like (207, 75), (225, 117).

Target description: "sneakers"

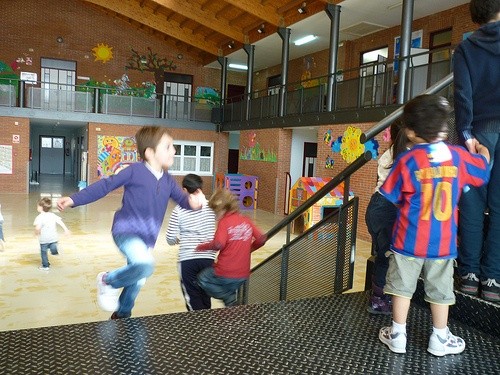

(479, 277), (500, 302)
(454, 273), (479, 294)
(39, 266), (50, 271)
(427, 330), (466, 357)
(379, 326), (407, 354)
(367, 295), (392, 315)
(97, 271), (119, 312)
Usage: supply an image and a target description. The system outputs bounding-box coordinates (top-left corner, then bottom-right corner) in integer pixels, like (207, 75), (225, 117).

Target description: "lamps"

(228, 44), (233, 48)
(257, 28), (264, 33)
(298, 7), (305, 14)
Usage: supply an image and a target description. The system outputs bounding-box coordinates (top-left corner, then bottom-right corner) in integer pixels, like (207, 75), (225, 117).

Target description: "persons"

(57, 125), (203, 321)
(366, 95), (491, 356)
(166, 173), (217, 311)
(33, 198), (70, 270)
(194, 189), (267, 307)
(454, 0), (500, 301)
